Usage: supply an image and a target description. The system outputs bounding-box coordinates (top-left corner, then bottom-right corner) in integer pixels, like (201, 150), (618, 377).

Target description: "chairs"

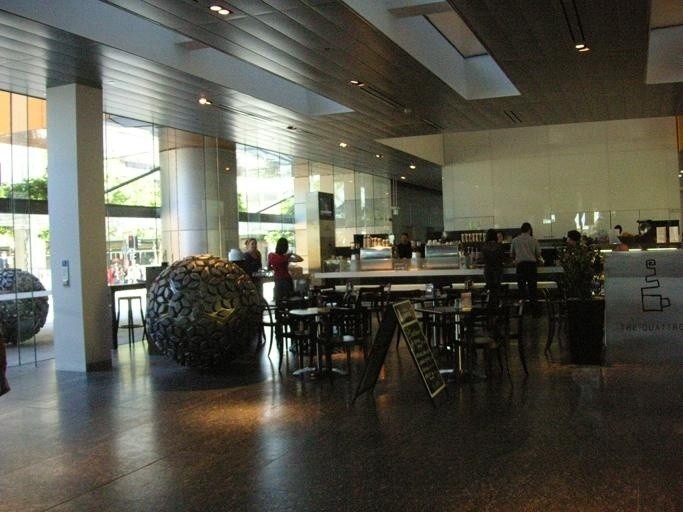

(257, 279), (577, 385)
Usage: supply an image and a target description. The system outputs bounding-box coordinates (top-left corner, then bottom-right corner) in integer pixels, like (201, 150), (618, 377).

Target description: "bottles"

(329, 233), (486, 269)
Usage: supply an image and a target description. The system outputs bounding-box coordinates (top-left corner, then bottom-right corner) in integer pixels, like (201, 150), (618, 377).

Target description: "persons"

(479, 223), (545, 319)
(565, 229), (587, 253)
(397, 232), (413, 260)
(243, 238), (263, 297)
(267, 237), (304, 319)
(107, 259), (142, 285)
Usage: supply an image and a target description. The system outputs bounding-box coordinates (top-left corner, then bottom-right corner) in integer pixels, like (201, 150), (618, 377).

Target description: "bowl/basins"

(227, 247), (244, 262)
(615, 236), (639, 248)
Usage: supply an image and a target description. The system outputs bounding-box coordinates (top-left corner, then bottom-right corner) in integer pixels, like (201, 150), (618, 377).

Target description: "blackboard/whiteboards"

(393, 299), (447, 399)
